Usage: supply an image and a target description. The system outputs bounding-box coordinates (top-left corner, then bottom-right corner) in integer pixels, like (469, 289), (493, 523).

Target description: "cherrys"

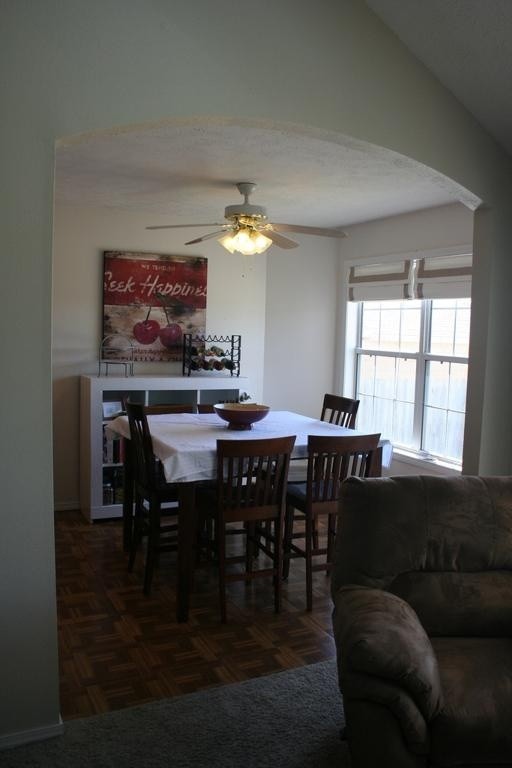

(131, 289), (182, 349)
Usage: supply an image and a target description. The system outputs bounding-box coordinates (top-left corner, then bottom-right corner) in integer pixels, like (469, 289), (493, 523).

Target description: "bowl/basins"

(214, 403), (270, 430)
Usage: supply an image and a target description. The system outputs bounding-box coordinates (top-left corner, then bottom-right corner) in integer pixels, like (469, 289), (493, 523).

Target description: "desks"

(103, 410), (393, 565)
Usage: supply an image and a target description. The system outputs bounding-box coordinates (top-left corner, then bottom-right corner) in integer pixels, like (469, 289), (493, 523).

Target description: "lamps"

(217, 228), (273, 256)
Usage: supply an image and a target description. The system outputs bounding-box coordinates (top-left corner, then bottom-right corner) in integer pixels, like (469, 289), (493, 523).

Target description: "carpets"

(0, 659), (345, 768)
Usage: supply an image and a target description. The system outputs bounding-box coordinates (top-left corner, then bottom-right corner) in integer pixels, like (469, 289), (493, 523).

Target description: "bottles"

(209, 358), (223, 369)
(198, 359), (213, 370)
(221, 358), (236, 370)
(184, 358), (200, 371)
(211, 346), (225, 357)
(186, 345), (199, 357)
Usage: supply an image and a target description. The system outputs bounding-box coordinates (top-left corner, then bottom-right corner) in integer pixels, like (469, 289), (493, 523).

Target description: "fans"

(146, 183), (348, 249)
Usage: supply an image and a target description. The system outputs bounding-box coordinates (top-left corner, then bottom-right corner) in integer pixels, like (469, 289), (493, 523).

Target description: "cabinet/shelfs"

(182, 334), (241, 377)
(80, 375), (249, 523)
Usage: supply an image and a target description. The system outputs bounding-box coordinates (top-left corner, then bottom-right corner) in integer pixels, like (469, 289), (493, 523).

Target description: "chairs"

(125, 403), (212, 593)
(252, 393), (360, 552)
(198, 404), (212, 413)
(284, 434), (385, 612)
(145, 402), (193, 413)
(177, 435), (296, 623)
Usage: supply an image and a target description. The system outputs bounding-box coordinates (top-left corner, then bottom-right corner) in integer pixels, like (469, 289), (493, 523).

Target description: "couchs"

(332, 476), (512, 768)
